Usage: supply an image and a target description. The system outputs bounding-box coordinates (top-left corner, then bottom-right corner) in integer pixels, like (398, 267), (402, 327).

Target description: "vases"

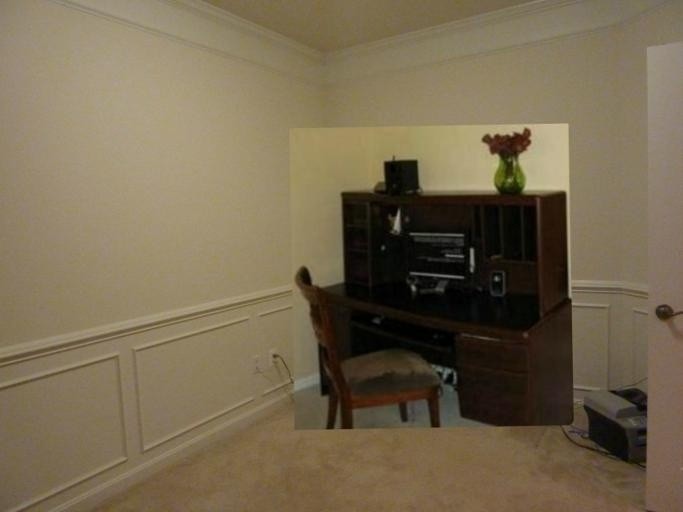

(493, 150), (527, 195)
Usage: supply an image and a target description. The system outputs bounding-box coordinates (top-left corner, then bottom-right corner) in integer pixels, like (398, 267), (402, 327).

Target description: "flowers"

(482, 126), (533, 189)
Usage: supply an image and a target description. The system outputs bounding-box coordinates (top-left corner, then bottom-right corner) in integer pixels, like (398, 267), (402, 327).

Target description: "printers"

(583, 387), (647, 465)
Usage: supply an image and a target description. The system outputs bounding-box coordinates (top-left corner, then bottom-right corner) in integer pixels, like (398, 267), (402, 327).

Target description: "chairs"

(293, 264), (443, 431)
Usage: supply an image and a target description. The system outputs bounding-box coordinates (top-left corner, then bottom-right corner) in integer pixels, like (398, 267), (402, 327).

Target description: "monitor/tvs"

(403, 225), (470, 297)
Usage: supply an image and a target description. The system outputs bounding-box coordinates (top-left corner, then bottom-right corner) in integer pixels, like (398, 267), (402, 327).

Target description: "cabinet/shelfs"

(338, 186), (571, 319)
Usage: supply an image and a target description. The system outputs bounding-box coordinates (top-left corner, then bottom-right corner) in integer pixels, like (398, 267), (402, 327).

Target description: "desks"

(317, 278), (576, 426)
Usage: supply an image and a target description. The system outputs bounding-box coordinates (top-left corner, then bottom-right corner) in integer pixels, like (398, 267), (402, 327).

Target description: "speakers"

(489, 269), (506, 298)
(384, 159), (419, 195)
